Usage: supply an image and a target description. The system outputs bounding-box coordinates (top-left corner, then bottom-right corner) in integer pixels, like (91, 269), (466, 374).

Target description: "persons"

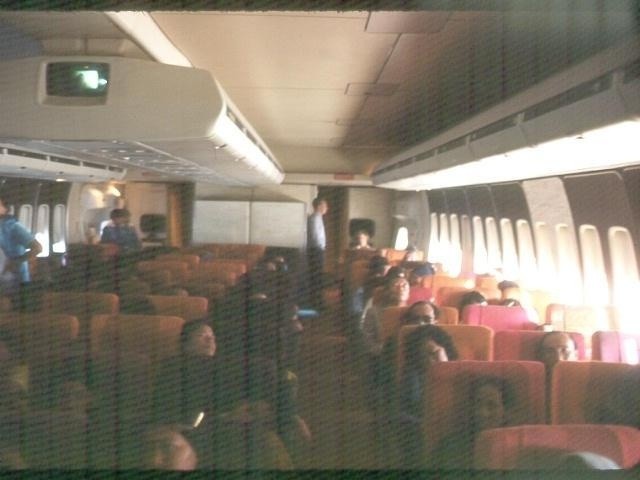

(99, 209), (133, 247)
(30, 365), (115, 465)
(305, 198), (332, 309)
(348, 229), (377, 249)
(1, 325), (33, 410)
(139, 254), (308, 470)
(353, 244), (576, 470)
(0, 188), (42, 309)
